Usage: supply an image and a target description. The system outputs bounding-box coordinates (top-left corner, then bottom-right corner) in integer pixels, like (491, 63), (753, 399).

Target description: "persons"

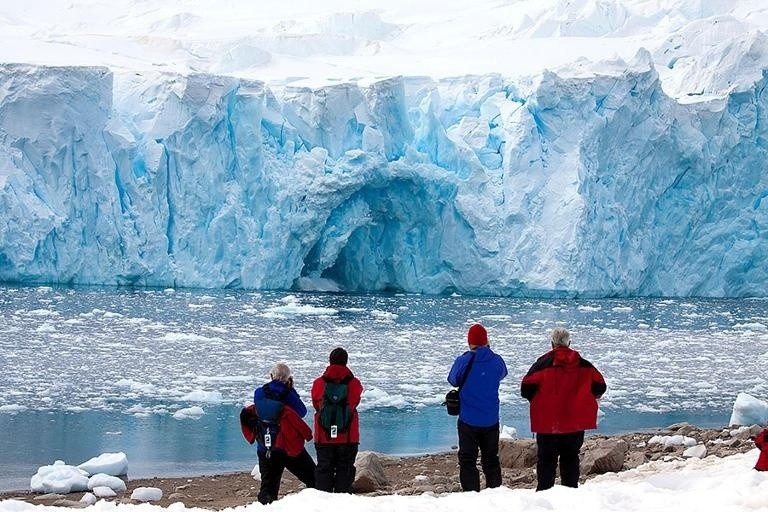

(447, 324), (508, 493)
(311, 348), (364, 494)
(254, 364), (319, 505)
(521, 327), (607, 493)
(750, 426), (768, 472)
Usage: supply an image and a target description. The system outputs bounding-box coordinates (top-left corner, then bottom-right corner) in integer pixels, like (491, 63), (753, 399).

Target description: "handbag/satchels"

(442, 389), (460, 415)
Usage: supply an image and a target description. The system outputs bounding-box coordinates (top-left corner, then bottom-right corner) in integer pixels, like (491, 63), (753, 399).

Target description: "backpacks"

(318, 372), (356, 436)
(254, 383), (292, 450)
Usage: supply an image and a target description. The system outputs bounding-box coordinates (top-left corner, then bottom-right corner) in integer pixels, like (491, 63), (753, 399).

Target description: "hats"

(467, 324), (488, 346)
